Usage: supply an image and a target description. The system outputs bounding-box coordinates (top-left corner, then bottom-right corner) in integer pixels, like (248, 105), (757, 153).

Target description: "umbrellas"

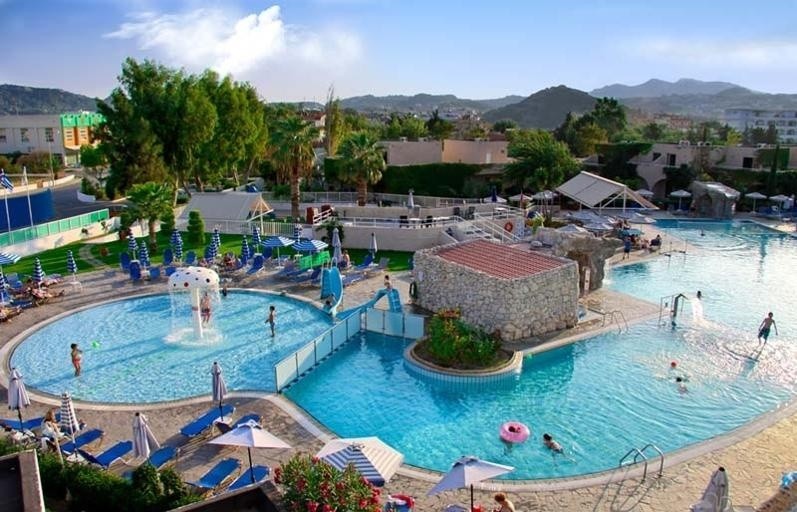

(0, 169), (13, 191)
(0, 249), (78, 302)
(424, 456), (515, 512)
(634, 188), (788, 218)
(127, 226), (343, 274)
(8, 368), (30, 424)
(60, 393), (80, 459)
(132, 361), (405, 493)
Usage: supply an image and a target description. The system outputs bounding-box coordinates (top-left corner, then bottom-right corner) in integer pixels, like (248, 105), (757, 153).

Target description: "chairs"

(46, 429), (104, 463)
(182, 457), (243, 498)
(0, 412), (61, 433)
(119, 245), (391, 290)
(78, 439), (135, 472)
(213, 463), (271, 498)
(759, 206), (797, 223)
(214, 414), (264, 440)
(0, 272), (82, 321)
(30, 430), (71, 453)
(123, 445), (181, 484)
(383, 494), (415, 512)
(178, 402), (236, 438)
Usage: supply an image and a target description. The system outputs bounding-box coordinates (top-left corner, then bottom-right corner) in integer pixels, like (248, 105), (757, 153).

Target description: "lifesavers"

(506, 222), (513, 231)
(500, 422), (530, 443)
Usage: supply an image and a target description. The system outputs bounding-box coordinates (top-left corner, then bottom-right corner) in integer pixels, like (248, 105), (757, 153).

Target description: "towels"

(40, 437), (50, 452)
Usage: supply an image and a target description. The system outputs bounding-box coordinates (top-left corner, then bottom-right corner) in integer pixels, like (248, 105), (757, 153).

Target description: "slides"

(322, 266), (344, 315)
(336, 288), (387, 321)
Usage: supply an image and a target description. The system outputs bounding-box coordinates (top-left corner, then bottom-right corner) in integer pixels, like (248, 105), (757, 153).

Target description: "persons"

(265, 306), (275, 336)
(617, 219), (661, 259)
(509, 425), (522, 434)
(343, 250), (350, 267)
(495, 494), (515, 512)
(677, 377), (681, 382)
(757, 312), (778, 344)
(544, 434), (577, 468)
(41, 412), (65, 442)
(200, 292), (210, 328)
(669, 363), (676, 372)
(70, 344), (82, 376)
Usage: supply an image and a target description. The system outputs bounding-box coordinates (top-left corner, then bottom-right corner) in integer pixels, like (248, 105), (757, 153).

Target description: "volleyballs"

(671, 361), (677, 367)
